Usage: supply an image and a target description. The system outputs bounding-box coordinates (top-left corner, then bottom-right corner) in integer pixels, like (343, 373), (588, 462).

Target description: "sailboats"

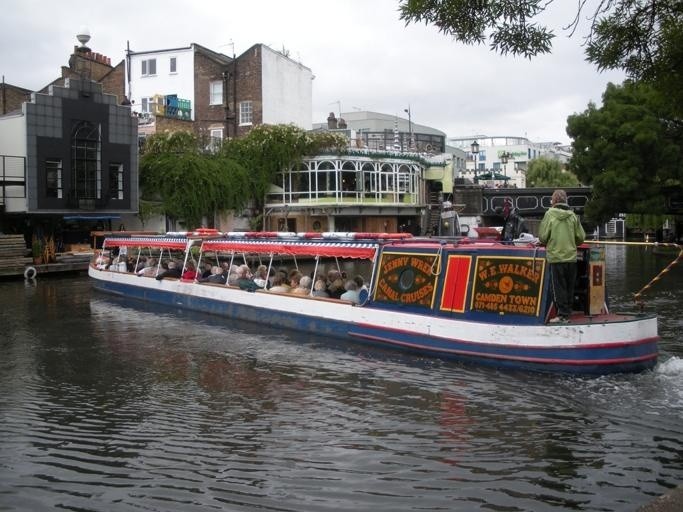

(89, 231), (659, 376)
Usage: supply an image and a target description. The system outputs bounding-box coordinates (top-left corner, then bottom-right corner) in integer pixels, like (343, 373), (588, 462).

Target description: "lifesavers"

(24, 267), (37, 280)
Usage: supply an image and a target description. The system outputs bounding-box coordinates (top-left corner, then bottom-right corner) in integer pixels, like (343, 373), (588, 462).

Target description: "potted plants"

(32, 240), (43, 264)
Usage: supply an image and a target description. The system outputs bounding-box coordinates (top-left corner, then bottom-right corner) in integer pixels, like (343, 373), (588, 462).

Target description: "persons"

(493, 184), (498, 189)
(95, 253), (369, 305)
(538, 190), (586, 323)
(662, 217), (672, 243)
(513, 184), (518, 189)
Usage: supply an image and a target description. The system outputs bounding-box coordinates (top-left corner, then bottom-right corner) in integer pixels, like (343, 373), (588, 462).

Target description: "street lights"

(500, 152), (510, 189)
(471, 141), (479, 184)
(405, 106), (416, 150)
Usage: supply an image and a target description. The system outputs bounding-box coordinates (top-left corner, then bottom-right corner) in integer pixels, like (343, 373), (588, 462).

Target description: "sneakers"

(549, 317), (569, 323)
